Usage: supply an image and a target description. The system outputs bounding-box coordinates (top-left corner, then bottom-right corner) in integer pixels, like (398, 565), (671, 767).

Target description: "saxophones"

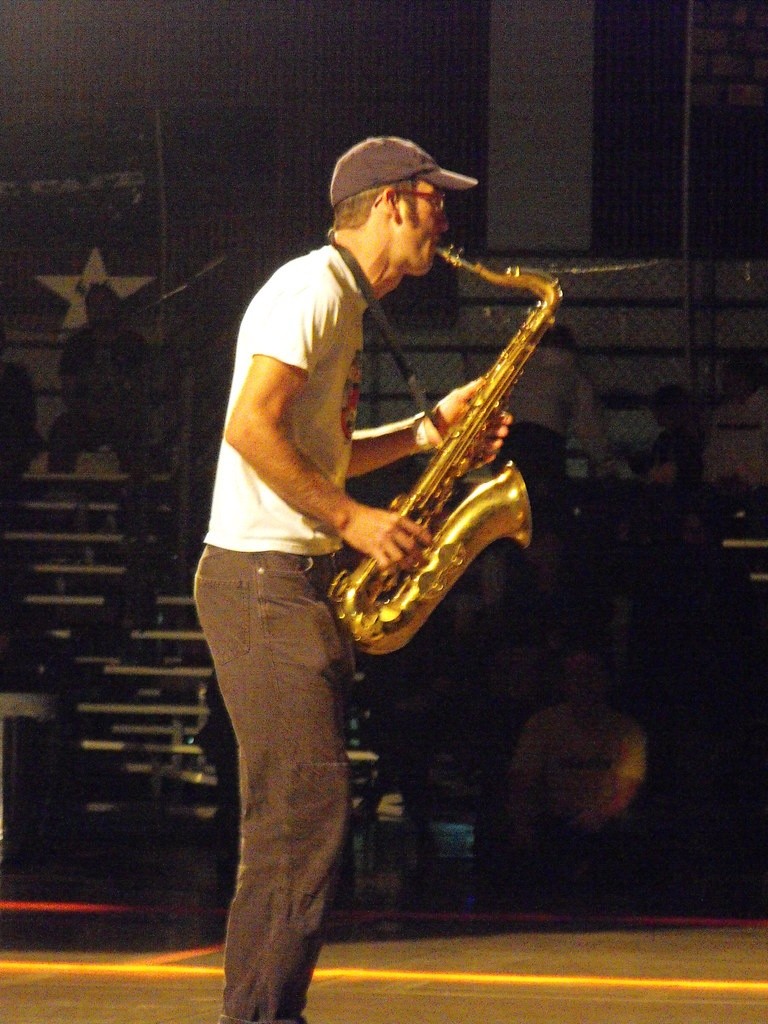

(328, 241), (565, 658)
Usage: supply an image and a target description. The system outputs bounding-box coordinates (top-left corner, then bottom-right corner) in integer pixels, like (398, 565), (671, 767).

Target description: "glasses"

(375, 187), (447, 216)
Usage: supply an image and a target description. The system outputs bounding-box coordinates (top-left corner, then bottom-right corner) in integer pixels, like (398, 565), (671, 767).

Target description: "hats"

(330, 136), (479, 207)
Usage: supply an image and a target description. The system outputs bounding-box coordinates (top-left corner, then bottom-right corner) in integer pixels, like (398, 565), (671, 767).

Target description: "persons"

(187, 134), (512, 1024)
(0, 288), (767, 874)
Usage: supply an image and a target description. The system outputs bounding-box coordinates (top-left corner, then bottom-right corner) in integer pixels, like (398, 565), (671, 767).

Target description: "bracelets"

(412, 412), (432, 453)
